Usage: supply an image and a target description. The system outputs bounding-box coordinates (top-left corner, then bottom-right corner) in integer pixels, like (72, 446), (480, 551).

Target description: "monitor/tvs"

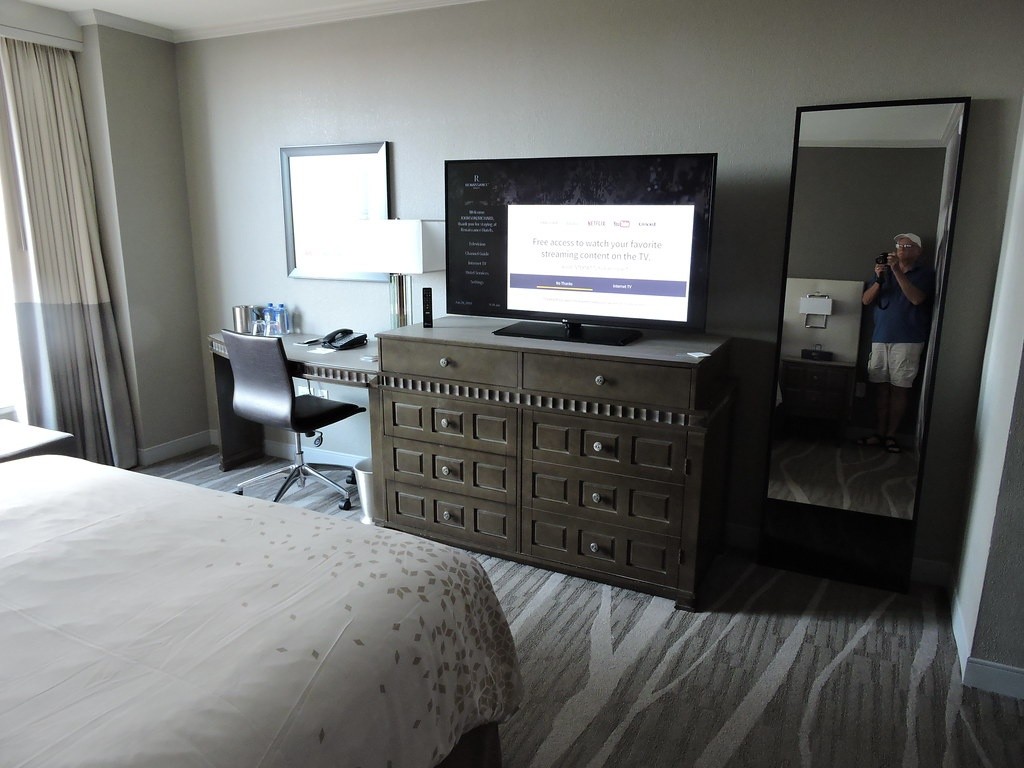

(444, 152), (718, 347)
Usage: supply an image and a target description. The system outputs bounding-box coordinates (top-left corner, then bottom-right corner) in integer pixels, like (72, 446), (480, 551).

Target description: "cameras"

(875, 253), (889, 267)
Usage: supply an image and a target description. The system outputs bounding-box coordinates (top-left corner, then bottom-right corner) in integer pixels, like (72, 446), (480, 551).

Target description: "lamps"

(360, 216), (424, 325)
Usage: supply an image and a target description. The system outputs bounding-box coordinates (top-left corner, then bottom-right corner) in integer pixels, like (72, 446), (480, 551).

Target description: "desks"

(0, 418), (81, 462)
(208, 330), (379, 472)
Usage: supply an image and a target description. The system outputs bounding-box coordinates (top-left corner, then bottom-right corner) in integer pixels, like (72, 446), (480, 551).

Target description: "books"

(294, 339), (320, 346)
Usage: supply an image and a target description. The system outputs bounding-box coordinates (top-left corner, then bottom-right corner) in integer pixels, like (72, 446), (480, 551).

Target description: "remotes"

(422, 287), (432, 328)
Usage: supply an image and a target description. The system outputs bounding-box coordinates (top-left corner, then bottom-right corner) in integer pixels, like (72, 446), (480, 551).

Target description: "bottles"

(275, 303), (289, 335)
(263, 302), (276, 335)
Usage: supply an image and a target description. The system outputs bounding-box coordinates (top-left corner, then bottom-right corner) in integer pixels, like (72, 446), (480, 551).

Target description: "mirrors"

(755, 93), (970, 592)
(281, 140), (391, 283)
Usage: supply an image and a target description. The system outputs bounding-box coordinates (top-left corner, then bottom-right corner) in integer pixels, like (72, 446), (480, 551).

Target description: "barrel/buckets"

(233, 305), (252, 334)
(248, 305), (264, 333)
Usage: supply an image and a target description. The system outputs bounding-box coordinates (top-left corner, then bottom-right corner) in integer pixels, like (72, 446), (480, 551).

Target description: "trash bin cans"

(352, 458), (373, 524)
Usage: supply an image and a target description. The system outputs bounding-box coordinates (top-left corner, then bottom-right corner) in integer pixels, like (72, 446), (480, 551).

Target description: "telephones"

(320, 328), (368, 350)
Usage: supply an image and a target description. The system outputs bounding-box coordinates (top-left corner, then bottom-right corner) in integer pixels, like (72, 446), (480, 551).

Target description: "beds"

(0, 453), (525, 768)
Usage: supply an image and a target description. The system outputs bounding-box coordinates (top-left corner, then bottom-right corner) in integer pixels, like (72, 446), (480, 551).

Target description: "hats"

(894, 233), (922, 248)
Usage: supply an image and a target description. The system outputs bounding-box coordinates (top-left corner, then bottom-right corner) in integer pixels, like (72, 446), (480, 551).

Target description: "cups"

(266, 321), (281, 336)
(252, 320), (266, 336)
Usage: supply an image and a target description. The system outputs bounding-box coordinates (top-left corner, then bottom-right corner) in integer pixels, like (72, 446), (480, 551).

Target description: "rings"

(877, 268), (879, 269)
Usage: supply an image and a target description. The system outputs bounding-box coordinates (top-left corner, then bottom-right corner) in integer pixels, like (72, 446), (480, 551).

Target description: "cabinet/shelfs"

(366, 316), (738, 610)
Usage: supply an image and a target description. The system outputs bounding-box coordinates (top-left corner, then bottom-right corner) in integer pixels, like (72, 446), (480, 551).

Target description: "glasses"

(897, 244), (917, 248)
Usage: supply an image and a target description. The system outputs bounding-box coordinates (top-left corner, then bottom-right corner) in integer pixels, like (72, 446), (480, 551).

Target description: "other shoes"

(857, 434), (901, 453)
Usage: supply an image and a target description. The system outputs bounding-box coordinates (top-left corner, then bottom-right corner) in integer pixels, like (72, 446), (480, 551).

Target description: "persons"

(863, 233), (931, 452)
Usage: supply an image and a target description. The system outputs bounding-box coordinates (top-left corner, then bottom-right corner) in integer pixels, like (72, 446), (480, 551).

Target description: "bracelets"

(876, 277), (883, 284)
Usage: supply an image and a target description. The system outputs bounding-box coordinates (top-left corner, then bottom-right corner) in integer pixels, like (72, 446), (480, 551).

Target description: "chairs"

(220, 329), (366, 512)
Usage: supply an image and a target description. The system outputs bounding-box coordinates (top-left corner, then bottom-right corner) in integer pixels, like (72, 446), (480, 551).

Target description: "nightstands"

(773, 355), (858, 442)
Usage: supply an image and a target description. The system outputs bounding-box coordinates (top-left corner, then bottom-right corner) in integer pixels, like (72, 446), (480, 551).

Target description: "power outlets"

(315, 389), (329, 399)
(856, 383), (866, 399)
